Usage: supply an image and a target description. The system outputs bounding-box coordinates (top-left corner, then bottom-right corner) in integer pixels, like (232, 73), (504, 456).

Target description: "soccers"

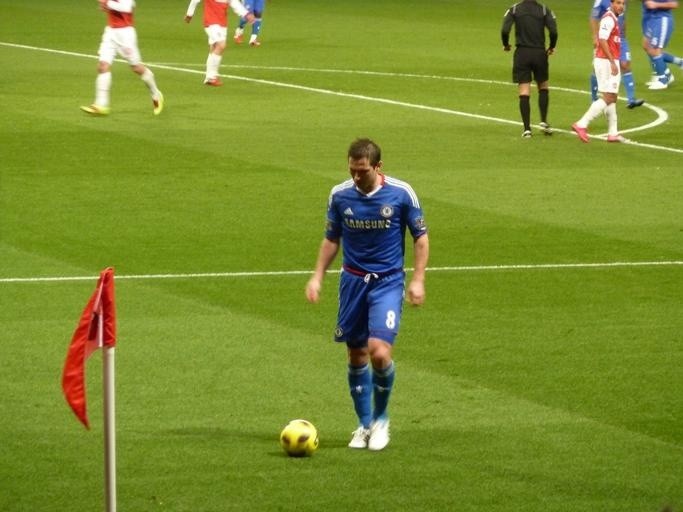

(280, 418), (319, 456)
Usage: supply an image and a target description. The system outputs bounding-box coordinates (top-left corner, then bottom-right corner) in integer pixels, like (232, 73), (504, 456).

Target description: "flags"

(59, 264), (118, 431)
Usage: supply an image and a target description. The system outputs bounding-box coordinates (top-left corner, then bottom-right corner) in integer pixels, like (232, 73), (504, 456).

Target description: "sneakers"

(249, 40), (260, 47)
(204, 78), (222, 86)
(648, 81), (667, 90)
(80, 105), (109, 115)
(233, 34), (242, 44)
(626, 99), (644, 110)
(521, 131), (530, 138)
(368, 418), (390, 450)
(539, 122), (552, 135)
(664, 70), (674, 84)
(571, 122), (589, 143)
(152, 92), (163, 115)
(607, 135), (627, 143)
(347, 425), (368, 448)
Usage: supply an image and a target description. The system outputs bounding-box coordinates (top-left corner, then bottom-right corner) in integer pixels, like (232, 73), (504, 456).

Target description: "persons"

(500, 0), (559, 138)
(571, 0), (626, 143)
(182, 0), (257, 87)
(232, 0), (265, 47)
(590, 0), (644, 109)
(640, 0), (683, 90)
(302, 136), (430, 453)
(78, 0), (166, 118)
